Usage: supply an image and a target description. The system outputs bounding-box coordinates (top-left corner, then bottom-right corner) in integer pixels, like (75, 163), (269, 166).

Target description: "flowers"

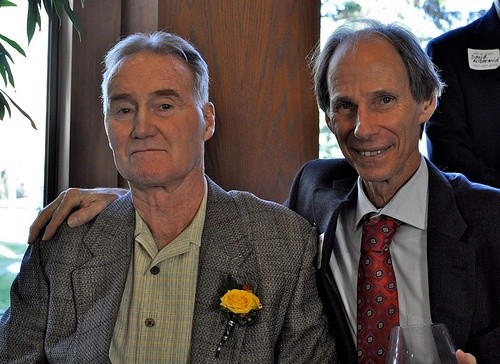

(216, 272), (262, 359)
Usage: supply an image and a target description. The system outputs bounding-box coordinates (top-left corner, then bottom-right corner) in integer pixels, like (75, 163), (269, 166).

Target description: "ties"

(355, 211), (407, 363)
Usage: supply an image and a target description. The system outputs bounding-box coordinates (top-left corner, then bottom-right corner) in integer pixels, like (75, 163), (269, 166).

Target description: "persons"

(1, 32), (339, 363)
(28, 19), (500, 363)
(426, 0), (500, 191)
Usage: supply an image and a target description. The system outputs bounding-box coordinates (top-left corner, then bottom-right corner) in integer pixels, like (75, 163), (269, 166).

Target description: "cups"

(387, 324), (457, 364)
